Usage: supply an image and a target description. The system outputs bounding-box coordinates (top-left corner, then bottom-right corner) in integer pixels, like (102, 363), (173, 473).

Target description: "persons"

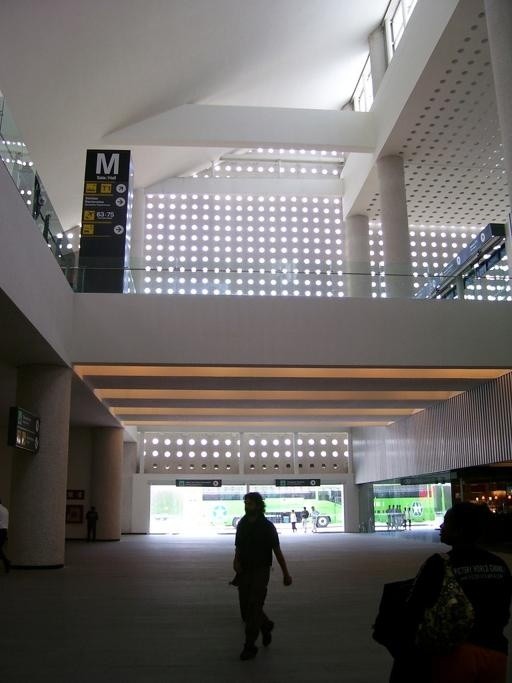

(301, 506), (309, 533)
(232, 492), (293, 661)
(0, 499), (12, 574)
(291, 509), (297, 533)
(373, 501), (510, 681)
(85, 506), (99, 542)
(309, 505), (320, 532)
(386, 504), (412, 529)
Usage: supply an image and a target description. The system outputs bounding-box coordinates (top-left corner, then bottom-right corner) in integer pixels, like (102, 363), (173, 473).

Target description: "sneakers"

(260, 619), (274, 647)
(239, 646), (258, 661)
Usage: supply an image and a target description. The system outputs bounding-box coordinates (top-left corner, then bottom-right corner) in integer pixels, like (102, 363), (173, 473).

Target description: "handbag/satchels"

(415, 551), (476, 659)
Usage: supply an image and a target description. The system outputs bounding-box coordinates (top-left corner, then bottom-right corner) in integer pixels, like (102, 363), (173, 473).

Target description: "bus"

(195, 482), (437, 528)
